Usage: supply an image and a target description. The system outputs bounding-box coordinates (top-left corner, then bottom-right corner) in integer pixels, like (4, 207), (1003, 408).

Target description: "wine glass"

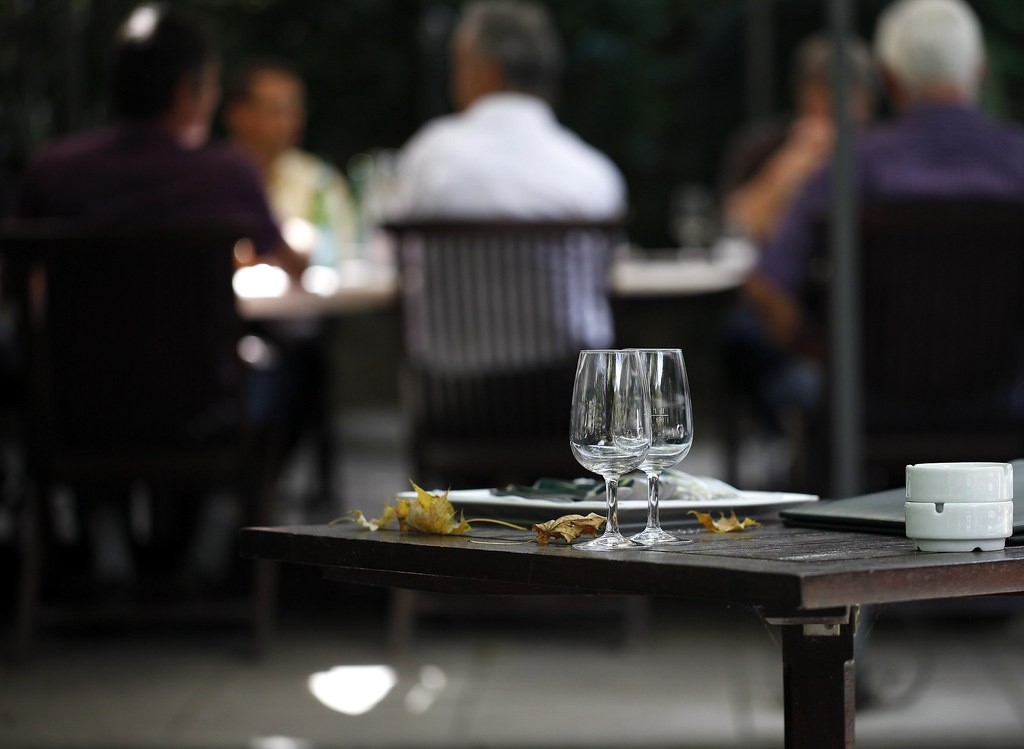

(569, 349), (694, 550)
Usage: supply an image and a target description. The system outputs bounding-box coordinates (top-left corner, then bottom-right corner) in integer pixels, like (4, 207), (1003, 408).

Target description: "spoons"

(489, 468), (710, 500)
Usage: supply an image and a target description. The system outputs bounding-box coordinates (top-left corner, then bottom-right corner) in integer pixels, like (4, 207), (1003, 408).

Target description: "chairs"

(381, 210), (649, 660)
(0, 217), (278, 664)
(802, 197), (1024, 632)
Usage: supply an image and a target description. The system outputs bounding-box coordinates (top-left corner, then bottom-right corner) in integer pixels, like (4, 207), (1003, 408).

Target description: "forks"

(533, 470), (746, 500)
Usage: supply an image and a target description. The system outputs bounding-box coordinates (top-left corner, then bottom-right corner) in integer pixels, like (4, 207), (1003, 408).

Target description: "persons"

(380, 9), (625, 439)
(721, 0), (1024, 511)
(0, 10), (359, 590)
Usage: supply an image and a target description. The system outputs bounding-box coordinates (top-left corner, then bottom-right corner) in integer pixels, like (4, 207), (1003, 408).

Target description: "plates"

(392, 479), (822, 526)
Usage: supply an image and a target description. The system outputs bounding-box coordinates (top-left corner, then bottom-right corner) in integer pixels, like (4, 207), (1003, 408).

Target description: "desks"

(236, 507), (1024, 749)
(247, 274), (745, 605)
